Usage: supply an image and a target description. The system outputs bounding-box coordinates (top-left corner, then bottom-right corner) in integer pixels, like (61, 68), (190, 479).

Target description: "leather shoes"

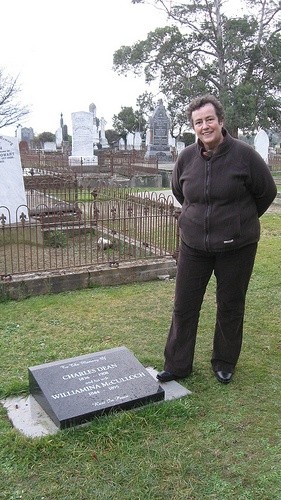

(156, 370), (189, 382)
(215, 370), (233, 382)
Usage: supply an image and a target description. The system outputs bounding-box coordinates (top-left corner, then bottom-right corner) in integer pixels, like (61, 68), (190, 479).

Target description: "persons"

(154, 93), (278, 383)
